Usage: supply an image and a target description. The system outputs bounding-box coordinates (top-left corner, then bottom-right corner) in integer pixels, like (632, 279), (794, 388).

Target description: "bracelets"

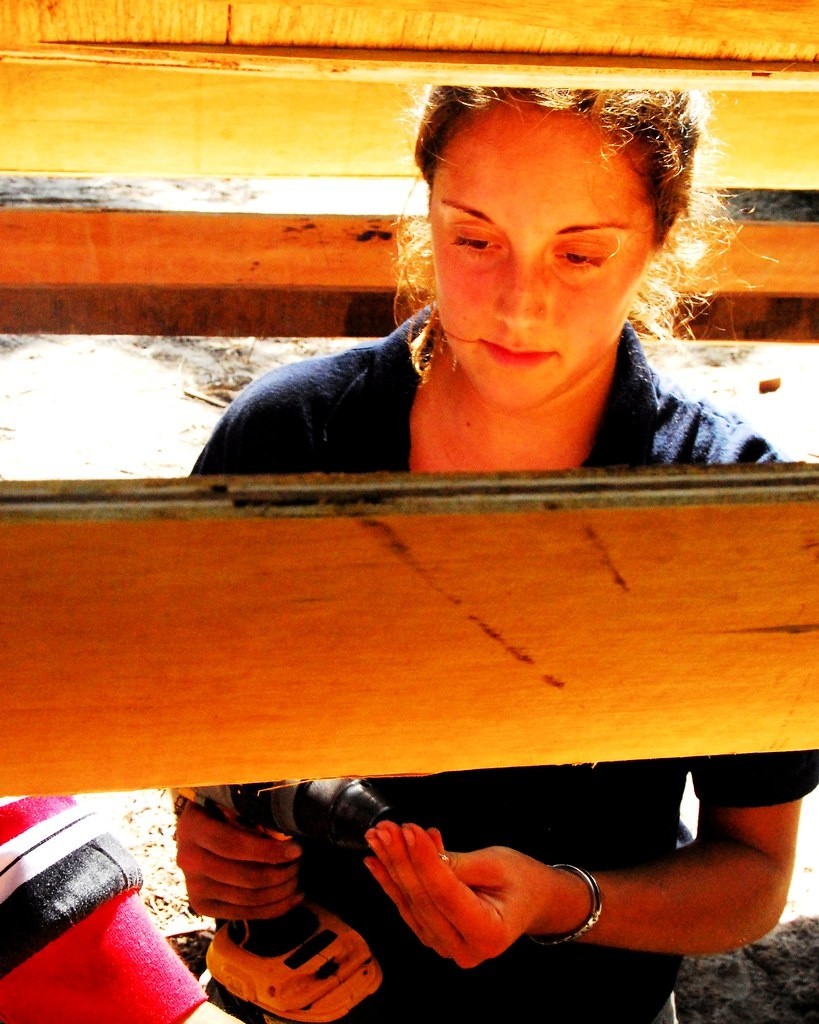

(526, 862), (601, 946)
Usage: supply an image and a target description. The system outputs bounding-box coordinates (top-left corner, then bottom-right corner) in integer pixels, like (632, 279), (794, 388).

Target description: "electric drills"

(171, 775), (450, 1024)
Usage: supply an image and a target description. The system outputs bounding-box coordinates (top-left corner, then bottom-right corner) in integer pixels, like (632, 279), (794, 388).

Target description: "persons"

(0, 796), (246, 1024)
(170, 81), (819, 1024)
